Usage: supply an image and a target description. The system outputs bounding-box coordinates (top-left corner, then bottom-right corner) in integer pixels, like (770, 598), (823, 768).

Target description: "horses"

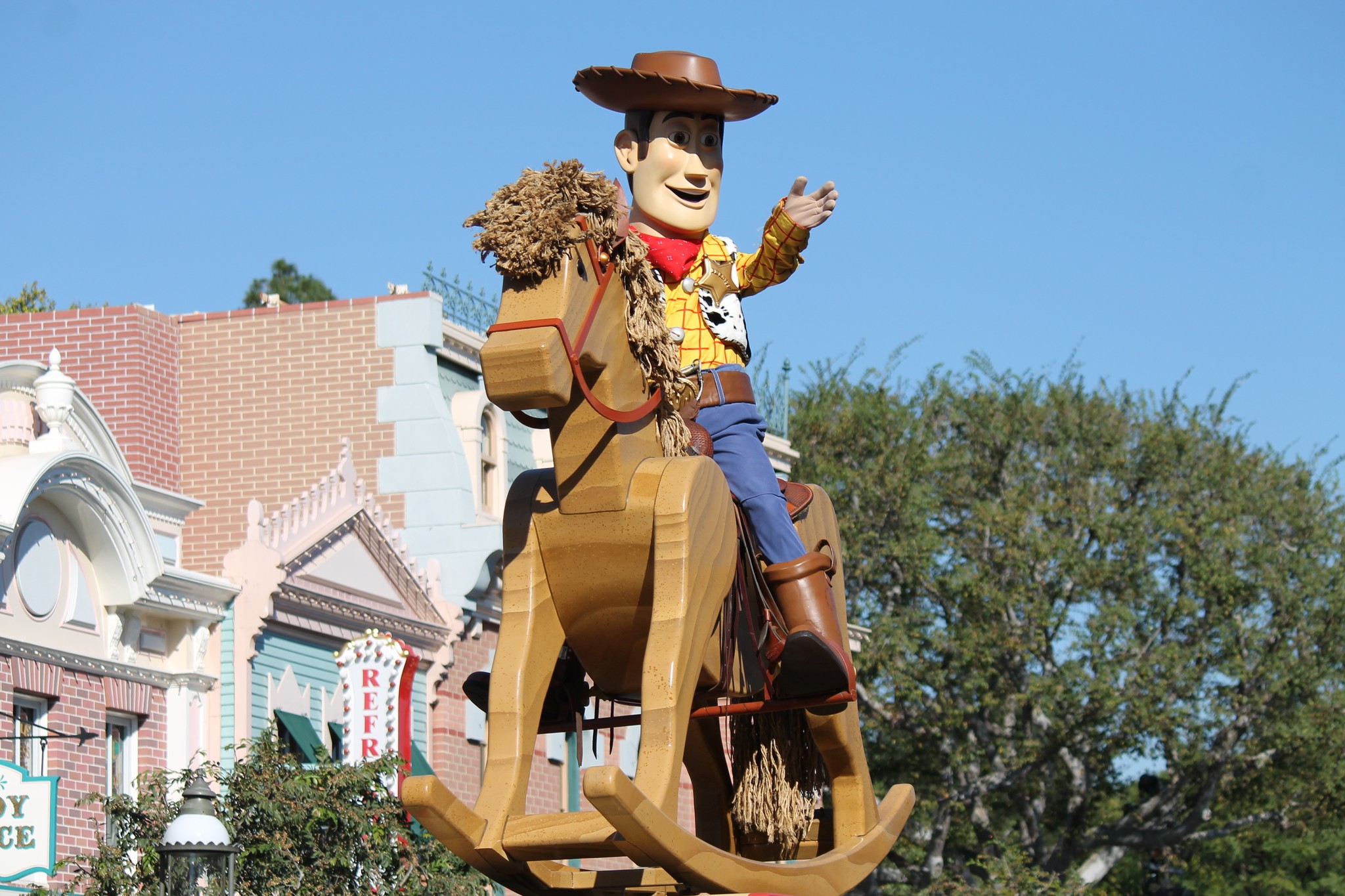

(474, 162), (883, 869)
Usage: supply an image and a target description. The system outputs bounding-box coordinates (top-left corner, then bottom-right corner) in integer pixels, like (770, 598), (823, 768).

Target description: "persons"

(460, 50), (856, 718)
